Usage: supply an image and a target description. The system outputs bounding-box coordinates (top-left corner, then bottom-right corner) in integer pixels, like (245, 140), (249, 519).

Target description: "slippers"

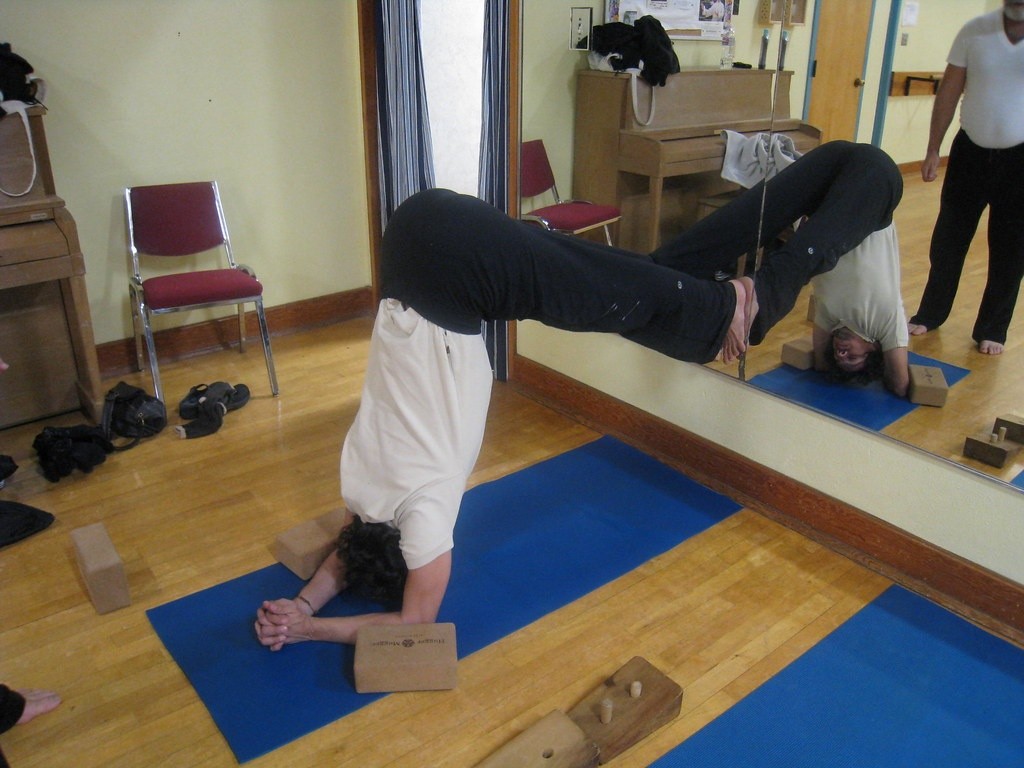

(178, 382), (251, 421)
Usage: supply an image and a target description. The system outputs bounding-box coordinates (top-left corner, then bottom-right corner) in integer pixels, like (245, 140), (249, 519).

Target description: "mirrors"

(511, 0), (1024, 491)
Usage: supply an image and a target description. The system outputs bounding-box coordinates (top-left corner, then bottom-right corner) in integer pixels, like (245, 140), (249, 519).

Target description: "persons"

(907, 0), (1024, 354)
(254, 189), (747, 652)
(0, 681), (62, 768)
(700, 0), (724, 22)
(649, 139), (910, 398)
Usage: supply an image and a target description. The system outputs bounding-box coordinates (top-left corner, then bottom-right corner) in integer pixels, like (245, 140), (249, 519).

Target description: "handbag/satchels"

(100, 381), (168, 452)
(1, 75), (49, 197)
(587, 50), (657, 126)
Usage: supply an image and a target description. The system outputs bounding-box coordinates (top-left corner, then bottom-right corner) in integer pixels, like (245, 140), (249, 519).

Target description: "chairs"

(124, 178), (281, 402)
(518, 139), (622, 245)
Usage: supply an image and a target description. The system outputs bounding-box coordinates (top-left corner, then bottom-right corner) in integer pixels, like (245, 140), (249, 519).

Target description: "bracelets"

(296, 595), (319, 616)
